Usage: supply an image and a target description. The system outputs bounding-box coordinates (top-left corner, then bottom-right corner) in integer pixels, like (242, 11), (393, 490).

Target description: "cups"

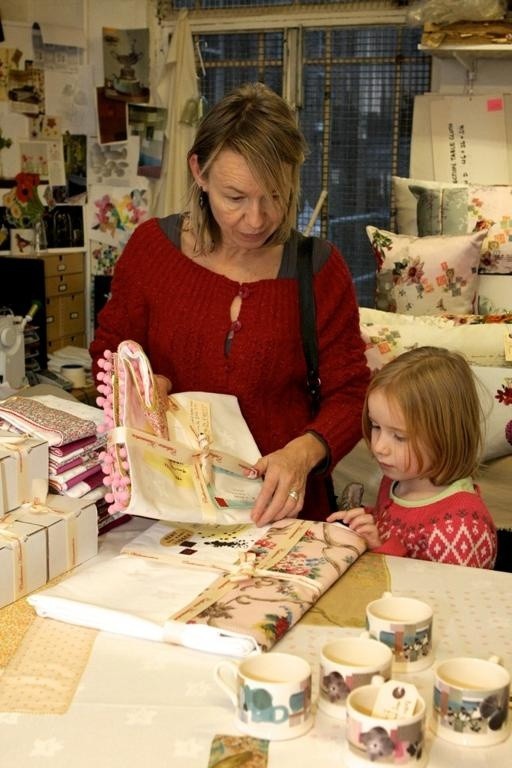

(343, 681), (429, 768)
(362, 590), (435, 676)
(313, 636), (394, 721)
(429, 652), (511, 752)
(60, 363), (88, 391)
(214, 649), (315, 742)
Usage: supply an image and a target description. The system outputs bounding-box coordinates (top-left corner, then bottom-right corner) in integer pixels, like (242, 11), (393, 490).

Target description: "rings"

(289, 492), (299, 502)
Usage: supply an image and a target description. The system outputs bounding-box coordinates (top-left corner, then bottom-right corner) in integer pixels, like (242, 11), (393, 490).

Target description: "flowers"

(3, 171), (46, 228)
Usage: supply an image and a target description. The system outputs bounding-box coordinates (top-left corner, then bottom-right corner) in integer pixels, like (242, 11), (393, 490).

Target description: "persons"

(326, 345), (498, 571)
(87, 82), (373, 528)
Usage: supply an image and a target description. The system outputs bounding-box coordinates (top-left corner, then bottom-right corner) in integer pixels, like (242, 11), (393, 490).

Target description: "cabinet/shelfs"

(0, 251), (88, 374)
